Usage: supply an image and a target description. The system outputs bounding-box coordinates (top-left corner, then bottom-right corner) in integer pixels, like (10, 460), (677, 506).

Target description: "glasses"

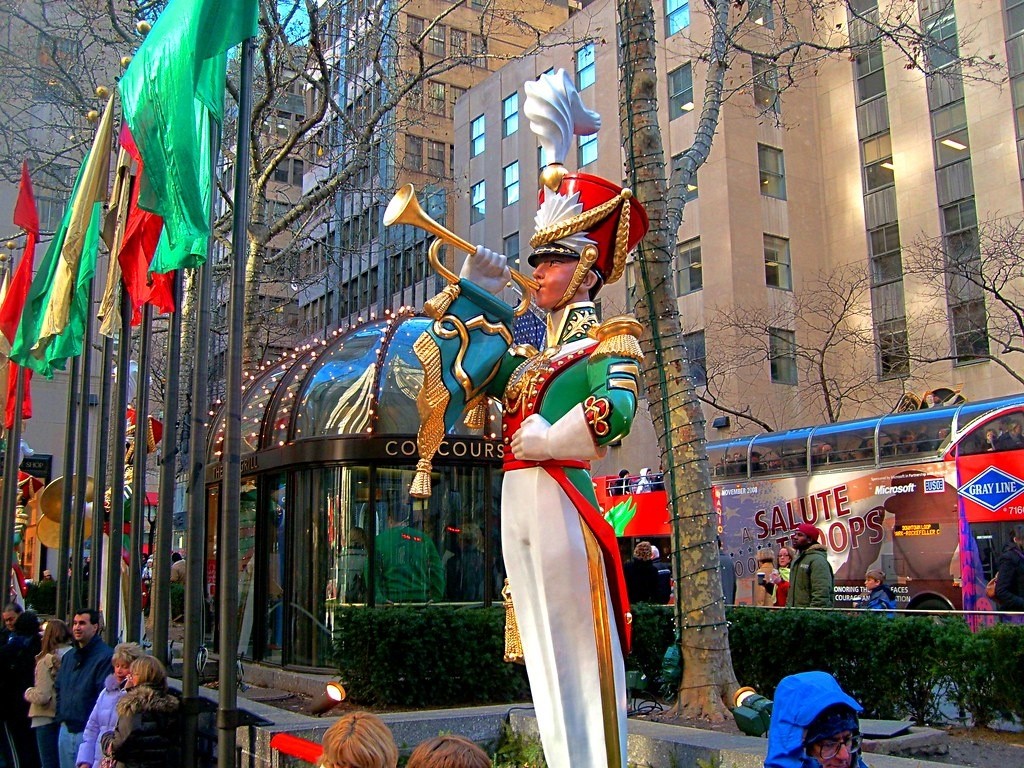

(815, 733), (863, 759)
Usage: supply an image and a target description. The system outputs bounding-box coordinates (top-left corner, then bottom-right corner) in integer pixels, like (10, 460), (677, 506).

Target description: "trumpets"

(382, 183), (540, 322)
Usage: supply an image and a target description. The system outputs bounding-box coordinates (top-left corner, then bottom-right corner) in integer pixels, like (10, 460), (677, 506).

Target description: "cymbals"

(40, 474), (94, 525)
(35, 514), (93, 550)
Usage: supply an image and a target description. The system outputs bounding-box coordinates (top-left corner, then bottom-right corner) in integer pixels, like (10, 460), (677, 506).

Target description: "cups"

(852, 598), (861, 608)
(757, 572), (765, 585)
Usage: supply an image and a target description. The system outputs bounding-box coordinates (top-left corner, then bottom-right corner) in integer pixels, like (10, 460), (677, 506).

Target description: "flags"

(0, 0), (260, 430)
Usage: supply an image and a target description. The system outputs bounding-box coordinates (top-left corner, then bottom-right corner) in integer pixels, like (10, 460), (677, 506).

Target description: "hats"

(807, 706), (858, 744)
(758, 548), (775, 560)
(797, 524), (821, 544)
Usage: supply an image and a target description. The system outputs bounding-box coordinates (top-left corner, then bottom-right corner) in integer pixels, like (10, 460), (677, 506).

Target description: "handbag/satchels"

(986, 571), (1001, 605)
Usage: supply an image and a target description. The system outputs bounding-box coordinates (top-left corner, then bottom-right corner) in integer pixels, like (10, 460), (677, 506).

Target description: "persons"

(764, 670), (868, 768)
(613, 463), (673, 605)
(0, 501), (486, 768)
(994, 524), (1024, 625)
(458, 68), (651, 768)
(752, 548), (777, 606)
(921, 389), (961, 409)
(784, 524), (834, 608)
(852, 570), (896, 619)
(714, 416), (1024, 476)
(716, 534), (737, 606)
(761, 547), (796, 608)
(70, 358), (165, 652)
(0, 440), (45, 612)
